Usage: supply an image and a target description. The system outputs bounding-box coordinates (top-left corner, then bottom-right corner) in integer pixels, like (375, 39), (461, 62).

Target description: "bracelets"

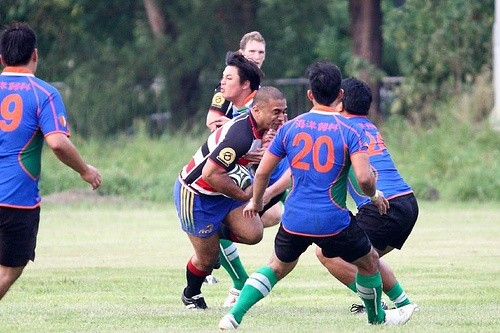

(369, 189), (379, 201)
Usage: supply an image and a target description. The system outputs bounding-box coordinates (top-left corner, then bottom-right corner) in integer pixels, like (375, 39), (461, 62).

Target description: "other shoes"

(203, 275), (218, 283)
(368, 303), (417, 325)
(350, 301), (388, 314)
(223, 295), (238, 307)
(219, 314), (239, 330)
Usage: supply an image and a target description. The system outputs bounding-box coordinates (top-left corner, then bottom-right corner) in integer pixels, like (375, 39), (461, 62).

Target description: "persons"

(0, 21), (101, 298)
(218, 60), (415, 332)
(334, 76), (420, 315)
(174, 86), (289, 310)
(205, 31), (278, 133)
(209, 50), (295, 309)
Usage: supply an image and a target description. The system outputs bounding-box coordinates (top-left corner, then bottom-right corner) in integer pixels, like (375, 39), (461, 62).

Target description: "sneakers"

(181, 287), (209, 310)
